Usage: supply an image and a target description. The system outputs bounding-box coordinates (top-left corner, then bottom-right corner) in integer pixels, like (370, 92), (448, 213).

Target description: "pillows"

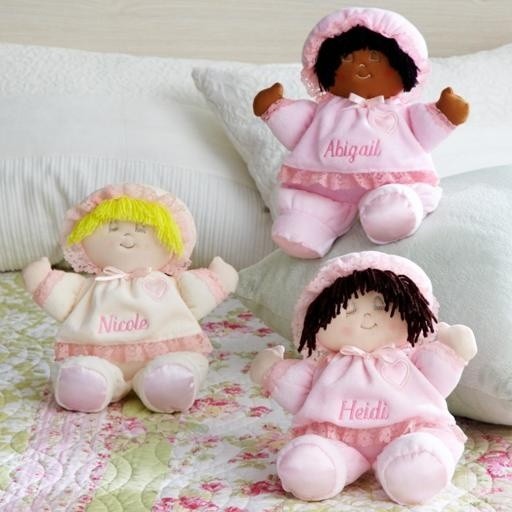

(2, 44), (277, 272)
(233, 164), (510, 425)
(190, 45), (511, 218)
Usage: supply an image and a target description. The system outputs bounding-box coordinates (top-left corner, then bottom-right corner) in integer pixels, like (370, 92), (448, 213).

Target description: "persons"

(252, 7), (470, 259)
(21, 181), (240, 414)
(246, 249), (479, 507)
